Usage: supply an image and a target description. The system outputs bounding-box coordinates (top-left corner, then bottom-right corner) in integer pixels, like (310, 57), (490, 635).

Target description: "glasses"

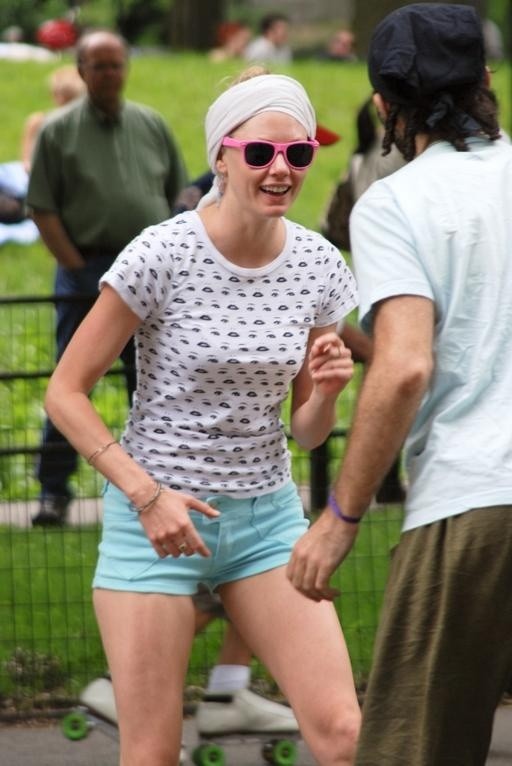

(82, 62), (125, 70)
(222, 136), (320, 170)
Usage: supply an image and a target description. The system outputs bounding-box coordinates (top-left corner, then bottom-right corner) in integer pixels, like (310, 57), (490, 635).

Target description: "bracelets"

(329, 490), (361, 524)
(128, 483), (162, 513)
(87, 441), (117, 465)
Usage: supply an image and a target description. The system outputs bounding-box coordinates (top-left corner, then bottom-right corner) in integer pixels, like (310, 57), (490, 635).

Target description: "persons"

(287, 0), (512, 766)
(76, 585), (299, 751)
(350, 97), (410, 203)
(206, 13), (293, 70)
(2, 18), (76, 62)
(0, 67), (88, 227)
(325, 100), (415, 503)
(27, 27), (187, 526)
(45, 70), (361, 766)
(315, 29), (358, 62)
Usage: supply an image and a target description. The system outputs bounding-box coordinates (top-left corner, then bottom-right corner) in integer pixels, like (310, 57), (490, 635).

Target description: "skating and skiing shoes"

(196, 688), (300, 739)
(76, 672), (119, 729)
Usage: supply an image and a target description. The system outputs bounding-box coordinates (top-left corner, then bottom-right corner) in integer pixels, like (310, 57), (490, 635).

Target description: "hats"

(367, 1), (486, 107)
(315, 126), (339, 145)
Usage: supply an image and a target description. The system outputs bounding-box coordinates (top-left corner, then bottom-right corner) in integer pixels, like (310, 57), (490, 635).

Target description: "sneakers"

(32, 492), (68, 526)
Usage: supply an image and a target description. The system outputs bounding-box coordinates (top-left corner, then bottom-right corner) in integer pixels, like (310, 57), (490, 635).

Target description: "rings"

(338, 346), (342, 360)
(178, 542), (188, 553)
